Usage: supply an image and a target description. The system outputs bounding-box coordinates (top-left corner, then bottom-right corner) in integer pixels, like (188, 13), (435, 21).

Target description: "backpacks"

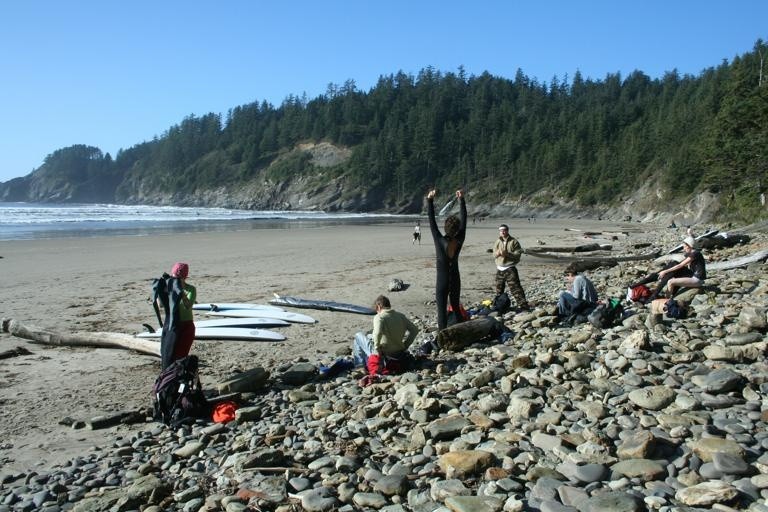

(151, 354), (202, 419)
(586, 297), (624, 330)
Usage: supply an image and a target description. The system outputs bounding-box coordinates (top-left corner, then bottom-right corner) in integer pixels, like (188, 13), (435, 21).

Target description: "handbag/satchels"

(365, 352), (416, 376)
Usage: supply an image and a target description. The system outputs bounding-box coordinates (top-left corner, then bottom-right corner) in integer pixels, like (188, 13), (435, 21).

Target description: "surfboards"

(705, 248), (768, 271)
(267, 292), (377, 314)
(204, 304), (316, 325)
(668, 230), (719, 254)
(192, 301), (286, 311)
(192, 317), (291, 329)
(135, 323), (286, 341)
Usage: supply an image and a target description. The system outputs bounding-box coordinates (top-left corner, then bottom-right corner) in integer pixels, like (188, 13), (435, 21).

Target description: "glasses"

(498, 229), (507, 232)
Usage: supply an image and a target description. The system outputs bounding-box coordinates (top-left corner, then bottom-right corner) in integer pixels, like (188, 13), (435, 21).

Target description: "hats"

(681, 236), (696, 249)
(171, 262), (190, 278)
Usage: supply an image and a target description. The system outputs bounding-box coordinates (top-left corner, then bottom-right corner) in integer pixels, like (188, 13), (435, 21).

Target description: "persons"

(425, 188), (468, 333)
(550, 264), (599, 322)
(488, 223), (530, 310)
(153, 261), (197, 363)
(411, 220), (422, 245)
(627, 236), (706, 302)
(350, 294), (420, 373)
